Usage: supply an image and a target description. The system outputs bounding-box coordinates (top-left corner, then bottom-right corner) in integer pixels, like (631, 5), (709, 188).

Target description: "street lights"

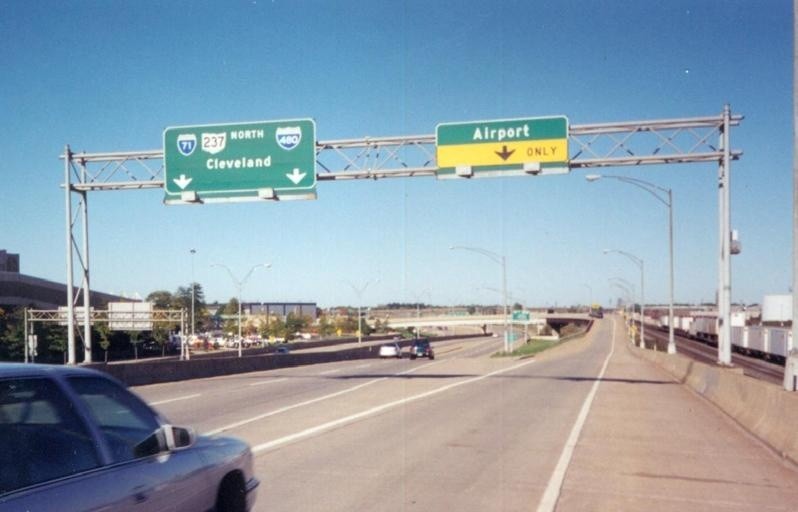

(189, 249), (196, 334)
(451, 245), (513, 354)
(585, 173), (676, 354)
(345, 277), (378, 344)
(210, 261), (271, 357)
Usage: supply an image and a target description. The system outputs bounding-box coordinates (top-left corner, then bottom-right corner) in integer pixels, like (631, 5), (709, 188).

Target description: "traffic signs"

(435, 115), (569, 169)
(512, 310), (530, 321)
(162, 118), (316, 196)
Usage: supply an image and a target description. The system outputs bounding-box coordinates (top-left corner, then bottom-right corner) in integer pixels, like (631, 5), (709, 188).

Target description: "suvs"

(410, 338), (434, 360)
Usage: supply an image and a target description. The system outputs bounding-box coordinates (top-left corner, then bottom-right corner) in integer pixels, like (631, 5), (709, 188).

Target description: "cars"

(378, 343), (403, 358)
(141, 327), (320, 353)
(0, 362), (259, 512)
